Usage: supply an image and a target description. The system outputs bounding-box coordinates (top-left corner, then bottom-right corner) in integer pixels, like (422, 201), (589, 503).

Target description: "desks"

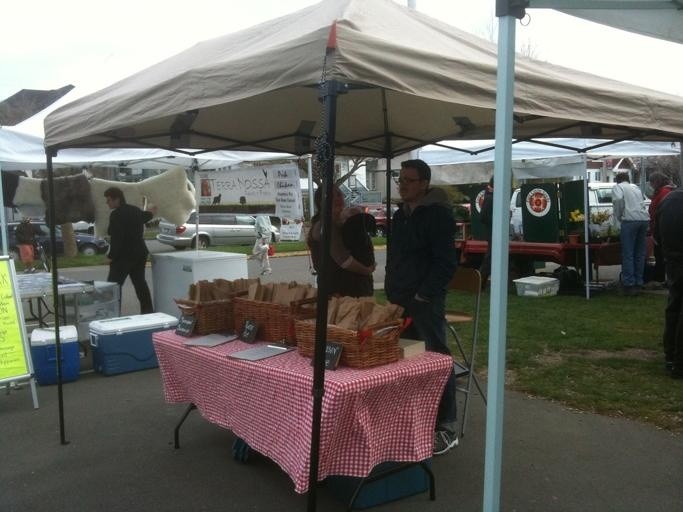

(152, 330), (453, 512)
(15, 273), (93, 356)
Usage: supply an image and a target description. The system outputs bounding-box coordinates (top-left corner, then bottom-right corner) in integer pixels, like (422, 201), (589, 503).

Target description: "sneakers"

(24, 269), (36, 273)
(432, 428), (459, 455)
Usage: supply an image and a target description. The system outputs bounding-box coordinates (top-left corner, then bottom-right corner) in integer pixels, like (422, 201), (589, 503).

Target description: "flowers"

(568, 209), (585, 234)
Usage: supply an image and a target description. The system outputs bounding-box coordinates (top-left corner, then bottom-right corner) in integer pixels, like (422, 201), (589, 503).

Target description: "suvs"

(357, 202), (400, 237)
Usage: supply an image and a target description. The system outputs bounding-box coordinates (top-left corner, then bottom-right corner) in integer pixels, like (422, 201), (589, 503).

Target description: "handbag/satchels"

(268, 246), (274, 256)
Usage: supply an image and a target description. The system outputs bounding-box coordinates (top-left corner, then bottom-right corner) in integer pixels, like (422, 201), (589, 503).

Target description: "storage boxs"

(512, 276), (560, 297)
(30, 325), (80, 386)
(89, 312), (179, 376)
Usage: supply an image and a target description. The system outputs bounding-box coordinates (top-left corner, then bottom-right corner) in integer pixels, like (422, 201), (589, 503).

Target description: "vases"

(568, 235), (580, 243)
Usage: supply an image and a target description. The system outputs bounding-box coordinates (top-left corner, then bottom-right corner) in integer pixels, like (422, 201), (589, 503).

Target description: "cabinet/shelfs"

(58, 280), (121, 356)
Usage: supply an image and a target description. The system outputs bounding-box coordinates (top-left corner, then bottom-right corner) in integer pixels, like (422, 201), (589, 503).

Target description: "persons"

(652, 189), (682, 378)
(15, 217), (48, 259)
(102, 186), (158, 314)
(477, 175), (493, 290)
(648, 170), (679, 288)
(247, 214), (275, 274)
(338, 205), (375, 297)
(385, 161), (461, 457)
(308, 182), (376, 299)
(612, 172), (651, 290)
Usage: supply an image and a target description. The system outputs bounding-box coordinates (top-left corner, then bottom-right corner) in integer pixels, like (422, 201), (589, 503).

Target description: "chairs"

(446, 267), (487, 438)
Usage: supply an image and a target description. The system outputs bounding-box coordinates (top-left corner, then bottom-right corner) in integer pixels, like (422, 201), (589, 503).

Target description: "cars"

(0, 222), (109, 258)
(156, 212), (281, 250)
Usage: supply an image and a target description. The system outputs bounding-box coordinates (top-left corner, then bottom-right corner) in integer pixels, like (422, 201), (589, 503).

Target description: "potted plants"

(589, 209), (621, 243)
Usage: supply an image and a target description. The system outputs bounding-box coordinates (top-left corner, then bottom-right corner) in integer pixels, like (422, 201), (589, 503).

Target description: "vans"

(510, 179), (653, 243)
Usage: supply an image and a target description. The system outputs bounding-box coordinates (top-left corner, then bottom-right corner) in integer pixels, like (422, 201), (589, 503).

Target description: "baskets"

(171, 291), (405, 368)
